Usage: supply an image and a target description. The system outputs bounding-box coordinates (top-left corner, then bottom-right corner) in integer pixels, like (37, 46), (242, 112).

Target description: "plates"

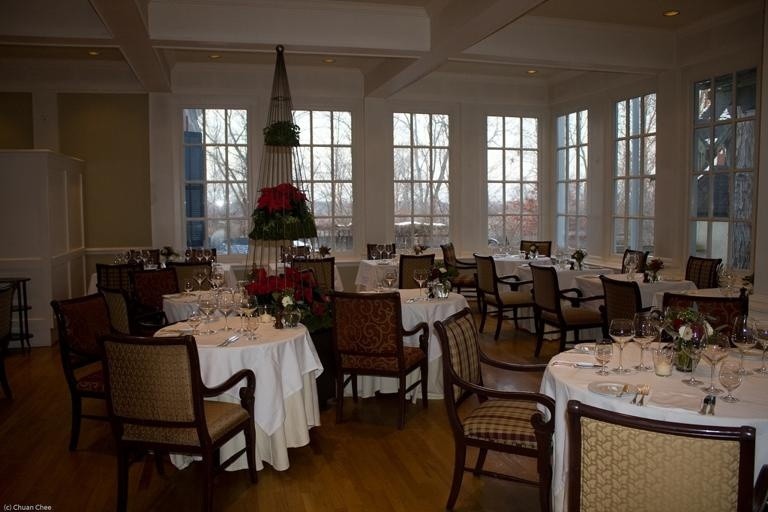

(588, 381), (636, 398)
(575, 343), (595, 354)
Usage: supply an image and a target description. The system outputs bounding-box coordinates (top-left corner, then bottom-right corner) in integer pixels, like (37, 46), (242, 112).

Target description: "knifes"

(552, 362), (607, 369)
(216, 335), (239, 347)
(696, 394), (716, 415)
(404, 297), (417, 303)
(617, 384), (629, 397)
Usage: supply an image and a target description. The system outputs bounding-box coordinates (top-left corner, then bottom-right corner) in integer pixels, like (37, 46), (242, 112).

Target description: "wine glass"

(623, 254), (640, 281)
(114, 248), (153, 269)
(718, 269), (744, 297)
(486, 240), (513, 259)
(681, 316), (768, 403)
(184, 267), (272, 341)
(371, 241), (399, 265)
(552, 246), (587, 270)
(596, 312), (659, 376)
(184, 247), (211, 263)
(374, 268), (397, 293)
(415, 270), (428, 300)
(401, 245), (444, 257)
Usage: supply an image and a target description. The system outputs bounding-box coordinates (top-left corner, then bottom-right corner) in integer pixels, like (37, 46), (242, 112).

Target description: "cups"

(519, 251), (526, 261)
(653, 346), (674, 376)
(436, 284), (449, 300)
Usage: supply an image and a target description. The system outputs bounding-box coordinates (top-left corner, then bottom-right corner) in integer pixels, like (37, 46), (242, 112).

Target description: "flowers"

(249, 184), (317, 240)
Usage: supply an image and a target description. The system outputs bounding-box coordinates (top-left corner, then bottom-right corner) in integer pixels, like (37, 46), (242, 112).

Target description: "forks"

(630, 384), (651, 407)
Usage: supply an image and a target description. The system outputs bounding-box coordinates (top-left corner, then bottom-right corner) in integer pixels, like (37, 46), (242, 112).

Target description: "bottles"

(274, 312), (285, 329)
